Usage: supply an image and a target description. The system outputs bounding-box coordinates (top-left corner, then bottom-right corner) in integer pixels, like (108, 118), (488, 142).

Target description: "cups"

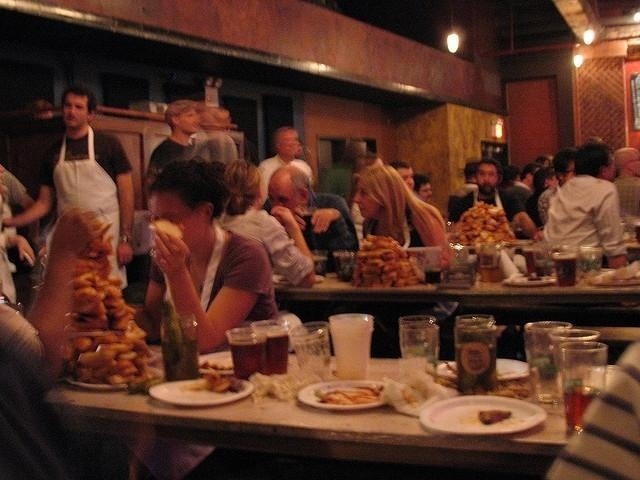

(550, 327), (601, 417)
(313, 239), (640, 289)
(525, 320), (572, 407)
(228, 326), (262, 379)
(331, 315), (375, 380)
(159, 316), (202, 380)
(289, 322), (331, 378)
(454, 316), (507, 394)
(559, 342), (608, 439)
(251, 319), (290, 375)
(399, 325), (439, 383)
(620, 220), (640, 242)
(399, 316), (437, 325)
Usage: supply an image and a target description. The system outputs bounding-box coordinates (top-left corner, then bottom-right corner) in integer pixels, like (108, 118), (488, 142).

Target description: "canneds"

(454, 314), (497, 391)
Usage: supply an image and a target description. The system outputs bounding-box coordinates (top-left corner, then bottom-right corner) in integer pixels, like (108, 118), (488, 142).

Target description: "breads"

(67, 237), (153, 385)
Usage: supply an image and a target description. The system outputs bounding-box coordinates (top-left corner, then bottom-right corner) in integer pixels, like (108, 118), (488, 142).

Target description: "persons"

(0, 143), (639, 479)
(144, 99), (199, 190)
(257, 124), (313, 198)
(0, 86), (136, 290)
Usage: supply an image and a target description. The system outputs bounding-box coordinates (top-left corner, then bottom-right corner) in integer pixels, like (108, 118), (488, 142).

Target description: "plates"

(65, 351), (164, 392)
(196, 351), (235, 375)
(298, 379), (390, 411)
(438, 357), (535, 382)
(150, 377), (255, 408)
(421, 393), (549, 438)
